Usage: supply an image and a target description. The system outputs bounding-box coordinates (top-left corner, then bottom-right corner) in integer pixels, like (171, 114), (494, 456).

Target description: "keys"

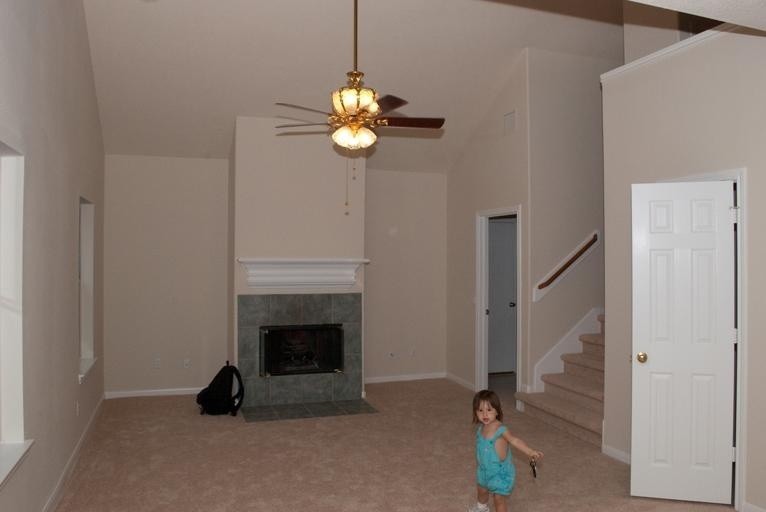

(529, 457), (536, 477)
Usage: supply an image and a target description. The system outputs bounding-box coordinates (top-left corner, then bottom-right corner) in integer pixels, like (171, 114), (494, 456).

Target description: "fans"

(271, 0), (446, 130)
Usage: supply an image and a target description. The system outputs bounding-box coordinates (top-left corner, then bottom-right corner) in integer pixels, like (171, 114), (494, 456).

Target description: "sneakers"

(467, 505), (490, 512)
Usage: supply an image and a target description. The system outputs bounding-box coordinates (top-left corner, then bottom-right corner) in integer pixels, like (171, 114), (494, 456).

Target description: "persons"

(473, 389), (543, 512)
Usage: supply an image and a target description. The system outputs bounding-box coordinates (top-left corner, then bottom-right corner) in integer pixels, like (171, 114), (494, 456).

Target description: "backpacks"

(196, 360), (245, 417)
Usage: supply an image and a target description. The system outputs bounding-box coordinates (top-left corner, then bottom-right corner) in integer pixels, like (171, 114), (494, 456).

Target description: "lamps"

(323, 67), (381, 153)
